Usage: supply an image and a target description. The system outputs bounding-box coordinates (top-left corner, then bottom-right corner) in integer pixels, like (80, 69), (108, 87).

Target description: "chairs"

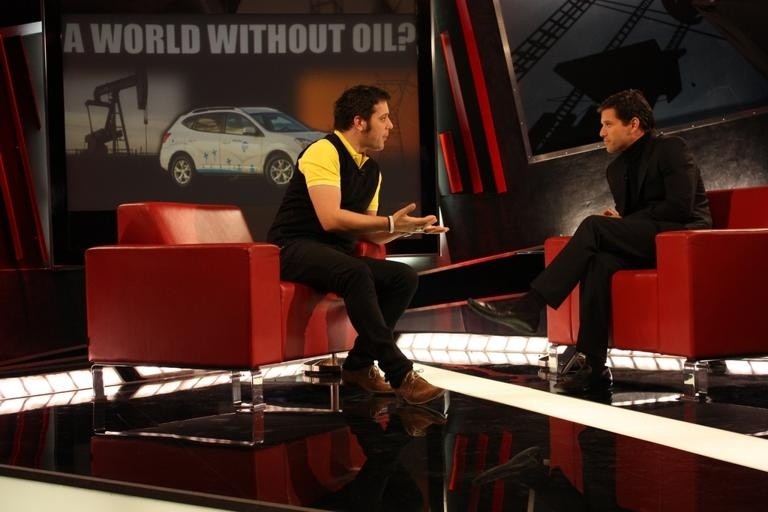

(80, 199), (357, 452)
(89, 426), (367, 512)
(538, 185), (768, 408)
(548, 402), (768, 512)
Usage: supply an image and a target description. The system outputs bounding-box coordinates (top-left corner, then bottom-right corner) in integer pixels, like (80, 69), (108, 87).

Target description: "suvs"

(157, 103), (331, 187)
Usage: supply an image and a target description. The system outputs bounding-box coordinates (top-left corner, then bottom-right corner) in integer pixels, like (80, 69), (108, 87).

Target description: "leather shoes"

(468, 298), (539, 333)
(554, 366), (614, 388)
(472, 447), (543, 486)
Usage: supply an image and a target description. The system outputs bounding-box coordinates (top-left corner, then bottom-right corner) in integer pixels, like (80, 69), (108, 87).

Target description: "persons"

(467, 89), (713, 395)
(264, 85), (451, 408)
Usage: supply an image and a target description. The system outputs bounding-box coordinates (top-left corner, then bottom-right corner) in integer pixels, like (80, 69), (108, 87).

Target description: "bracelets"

(388, 215), (396, 235)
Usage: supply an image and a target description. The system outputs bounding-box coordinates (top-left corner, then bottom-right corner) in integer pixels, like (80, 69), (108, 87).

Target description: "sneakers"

(342, 365), (444, 404)
(342, 393), (447, 437)
